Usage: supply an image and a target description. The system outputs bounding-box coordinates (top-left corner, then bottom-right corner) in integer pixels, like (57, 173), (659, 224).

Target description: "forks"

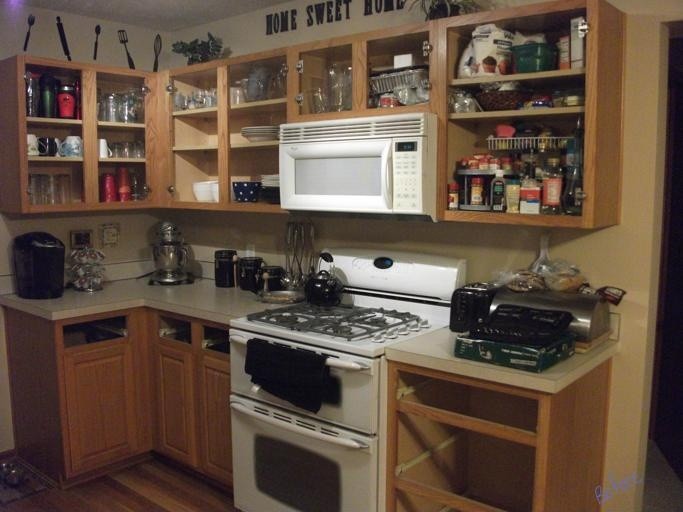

(20, 13), (36, 52)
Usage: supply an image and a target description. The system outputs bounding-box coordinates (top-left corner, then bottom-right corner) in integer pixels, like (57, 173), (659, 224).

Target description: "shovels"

(117, 30), (135, 70)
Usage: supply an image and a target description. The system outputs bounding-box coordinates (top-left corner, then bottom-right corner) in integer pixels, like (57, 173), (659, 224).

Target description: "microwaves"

(278, 113), (438, 218)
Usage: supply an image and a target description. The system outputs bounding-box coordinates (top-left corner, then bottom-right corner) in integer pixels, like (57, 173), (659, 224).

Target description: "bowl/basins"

(512, 43), (554, 74)
(471, 90), (530, 112)
(482, 63), (495, 74)
(0, 461), (26, 486)
(498, 66), (510, 75)
(232, 182), (261, 202)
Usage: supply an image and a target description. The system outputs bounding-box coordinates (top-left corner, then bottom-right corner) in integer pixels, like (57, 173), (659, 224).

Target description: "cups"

(175, 88), (217, 112)
(26, 133), (142, 159)
(306, 64), (352, 114)
(29, 165), (142, 205)
(95, 92), (137, 123)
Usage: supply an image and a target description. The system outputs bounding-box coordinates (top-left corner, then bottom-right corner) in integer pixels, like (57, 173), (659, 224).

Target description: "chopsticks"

(192, 181), (219, 202)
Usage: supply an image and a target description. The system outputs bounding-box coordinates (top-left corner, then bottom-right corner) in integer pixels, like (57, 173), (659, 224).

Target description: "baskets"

(368, 69), (428, 94)
(487, 134), (576, 150)
(476, 91), (532, 111)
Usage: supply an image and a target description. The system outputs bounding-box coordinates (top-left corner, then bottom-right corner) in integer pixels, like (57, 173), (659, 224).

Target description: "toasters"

(449, 281), (502, 333)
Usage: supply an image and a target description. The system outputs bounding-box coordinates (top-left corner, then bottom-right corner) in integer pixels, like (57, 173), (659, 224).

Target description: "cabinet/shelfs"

(386, 331), (611, 512)
(2, 306), (154, 491)
(169, 60), (223, 211)
(436, 0), (627, 231)
(0, 55), (93, 218)
(294, 19), (438, 124)
(224, 45), (298, 214)
(154, 310), (200, 477)
(201, 318), (232, 498)
(90, 65), (171, 212)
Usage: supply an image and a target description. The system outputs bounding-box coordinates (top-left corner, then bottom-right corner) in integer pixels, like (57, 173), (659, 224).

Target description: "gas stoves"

(228, 301), (449, 358)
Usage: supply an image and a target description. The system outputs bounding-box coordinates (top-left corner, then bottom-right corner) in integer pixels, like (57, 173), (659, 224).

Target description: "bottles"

(438, 116), (583, 219)
(529, 232), (553, 273)
(73, 80), (81, 120)
(54, 86), (75, 119)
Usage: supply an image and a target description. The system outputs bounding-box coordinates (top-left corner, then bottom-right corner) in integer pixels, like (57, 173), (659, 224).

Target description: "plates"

(259, 173), (279, 203)
(238, 126), (279, 142)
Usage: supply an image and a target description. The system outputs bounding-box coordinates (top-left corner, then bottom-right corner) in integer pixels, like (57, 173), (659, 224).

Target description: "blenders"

(152, 222), (190, 285)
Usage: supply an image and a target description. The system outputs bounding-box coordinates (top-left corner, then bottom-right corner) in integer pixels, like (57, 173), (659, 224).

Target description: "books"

(557, 36), (571, 70)
(569, 16), (584, 68)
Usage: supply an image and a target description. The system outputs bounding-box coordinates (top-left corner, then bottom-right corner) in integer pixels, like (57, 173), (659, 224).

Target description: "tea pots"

(302, 251), (342, 307)
(226, 58), (286, 104)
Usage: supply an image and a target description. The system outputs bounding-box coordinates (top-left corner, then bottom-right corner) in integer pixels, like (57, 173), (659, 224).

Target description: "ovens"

(227, 329), (383, 512)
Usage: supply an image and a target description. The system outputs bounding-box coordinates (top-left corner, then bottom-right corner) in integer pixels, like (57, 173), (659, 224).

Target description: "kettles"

(38, 73), (59, 118)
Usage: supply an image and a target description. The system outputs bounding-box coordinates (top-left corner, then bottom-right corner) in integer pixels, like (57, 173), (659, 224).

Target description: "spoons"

(90, 24), (100, 61)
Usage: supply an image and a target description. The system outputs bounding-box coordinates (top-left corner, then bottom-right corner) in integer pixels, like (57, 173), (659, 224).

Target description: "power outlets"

(97, 223), (123, 249)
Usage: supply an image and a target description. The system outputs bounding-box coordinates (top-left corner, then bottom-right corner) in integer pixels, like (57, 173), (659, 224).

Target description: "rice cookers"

(11, 231), (66, 300)
